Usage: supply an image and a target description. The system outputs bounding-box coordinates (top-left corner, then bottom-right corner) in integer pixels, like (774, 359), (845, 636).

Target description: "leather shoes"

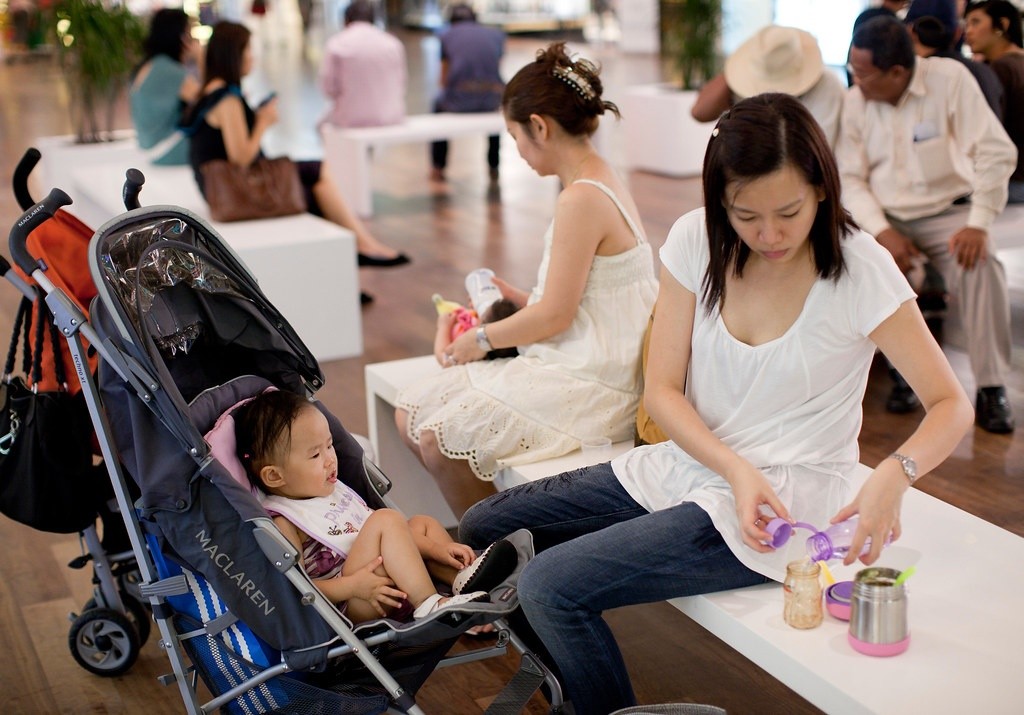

(975, 386), (1016, 436)
(886, 376), (921, 414)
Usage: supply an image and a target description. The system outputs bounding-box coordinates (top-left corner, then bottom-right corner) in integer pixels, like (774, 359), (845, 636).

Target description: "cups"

(847, 567), (912, 657)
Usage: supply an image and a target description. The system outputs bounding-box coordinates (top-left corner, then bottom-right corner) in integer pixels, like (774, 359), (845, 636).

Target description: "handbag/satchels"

(0, 284), (98, 534)
(200, 157), (305, 223)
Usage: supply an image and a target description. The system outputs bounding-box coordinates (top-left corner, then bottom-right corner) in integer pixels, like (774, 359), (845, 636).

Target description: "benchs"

(365, 351), (1024, 715)
(328, 111), (509, 219)
(73, 162), (364, 362)
(945, 204), (1024, 400)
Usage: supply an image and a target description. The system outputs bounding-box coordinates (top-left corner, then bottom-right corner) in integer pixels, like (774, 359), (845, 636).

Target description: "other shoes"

(452, 538), (518, 595)
(361, 292), (374, 305)
(413, 591), (490, 621)
(358, 252), (409, 267)
(428, 169), (445, 182)
(490, 168), (500, 181)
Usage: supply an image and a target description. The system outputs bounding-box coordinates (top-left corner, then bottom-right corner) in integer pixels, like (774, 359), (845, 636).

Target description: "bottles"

(464, 268), (504, 319)
(783, 559), (823, 630)
(763, 516), (893, 562)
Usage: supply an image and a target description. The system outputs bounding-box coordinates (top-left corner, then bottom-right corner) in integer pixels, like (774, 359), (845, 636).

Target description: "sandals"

(463, 623), (499, 641)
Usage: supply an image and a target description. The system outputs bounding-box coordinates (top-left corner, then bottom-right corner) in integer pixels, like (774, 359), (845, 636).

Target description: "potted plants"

(625, 0), (731, 180)
(21, 0), (151, 206)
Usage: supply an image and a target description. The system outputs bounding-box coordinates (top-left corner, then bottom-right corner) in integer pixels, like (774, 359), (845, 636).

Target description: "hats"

(723, 25), (823, 98)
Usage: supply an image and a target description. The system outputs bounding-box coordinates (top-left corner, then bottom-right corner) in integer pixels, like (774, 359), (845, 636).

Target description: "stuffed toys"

(432, 293), (480, 343)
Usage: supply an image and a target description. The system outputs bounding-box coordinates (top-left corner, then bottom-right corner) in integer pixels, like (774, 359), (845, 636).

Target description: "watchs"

(476, 324), (493, 352)
(889, 453), (917, 485)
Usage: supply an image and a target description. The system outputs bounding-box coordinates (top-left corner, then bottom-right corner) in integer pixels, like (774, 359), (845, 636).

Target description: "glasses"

(846, 62), (883, 86)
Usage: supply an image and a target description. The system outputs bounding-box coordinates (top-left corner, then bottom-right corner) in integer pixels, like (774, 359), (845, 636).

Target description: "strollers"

(0, 147), (570, 715)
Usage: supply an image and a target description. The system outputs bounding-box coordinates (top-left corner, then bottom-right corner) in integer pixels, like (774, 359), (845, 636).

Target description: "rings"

(448, 356), (456, 365)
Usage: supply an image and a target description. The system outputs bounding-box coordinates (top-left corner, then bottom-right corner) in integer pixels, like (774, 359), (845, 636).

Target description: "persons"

(393, 42), (663, 638)
(129, 0), (414, 305)
(231, 391), (491, 630)
(456, 92), (975, 715)
(434, 297), (521, 369)
(426, 4), (507, 183)
(691, 0), (1024, 436)
